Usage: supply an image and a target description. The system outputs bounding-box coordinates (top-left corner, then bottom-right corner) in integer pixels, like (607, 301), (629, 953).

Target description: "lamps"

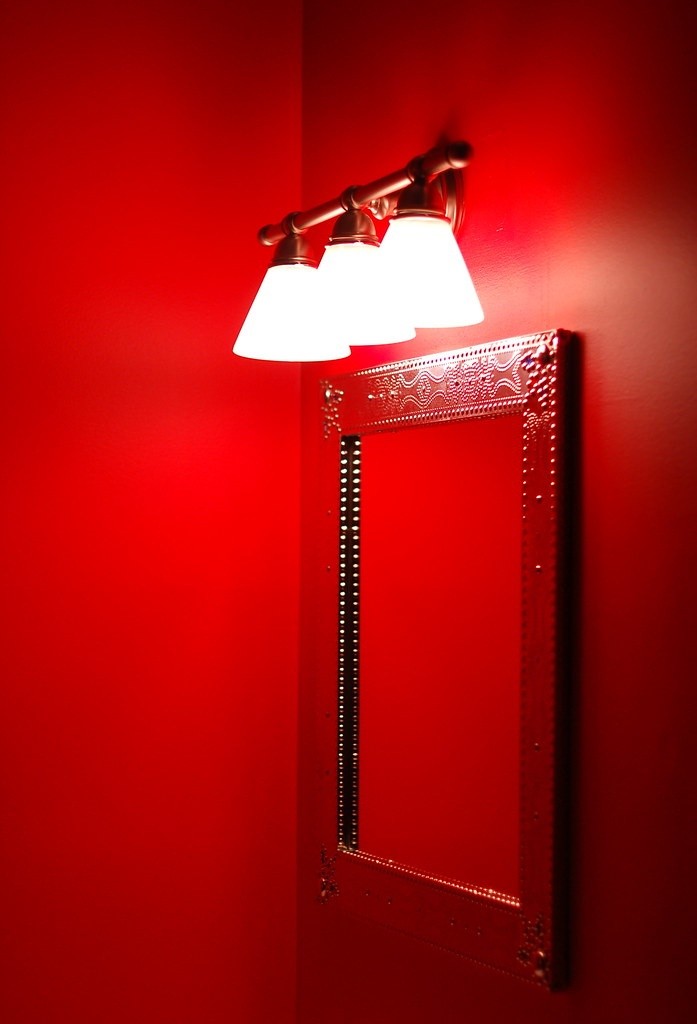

(232, 142), (486, 362)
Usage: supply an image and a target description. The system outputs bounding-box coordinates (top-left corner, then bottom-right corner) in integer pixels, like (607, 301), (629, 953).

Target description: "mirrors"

(315, 325), (572, 984)
